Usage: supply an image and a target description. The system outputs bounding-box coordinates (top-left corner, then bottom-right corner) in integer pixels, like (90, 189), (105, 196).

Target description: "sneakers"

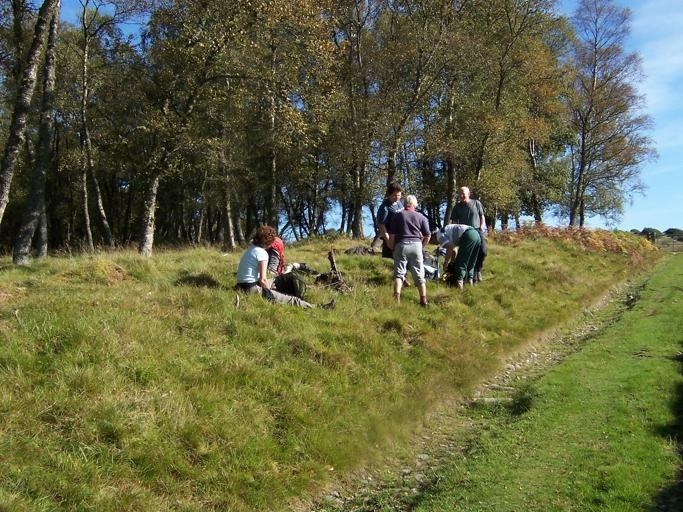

(322, 299), (335, 310)
(403, 280), (410, 288)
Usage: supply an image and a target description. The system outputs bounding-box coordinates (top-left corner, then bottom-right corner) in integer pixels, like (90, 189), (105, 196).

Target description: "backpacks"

(273, 272), (306, 299)
(442, 262), (458, 287)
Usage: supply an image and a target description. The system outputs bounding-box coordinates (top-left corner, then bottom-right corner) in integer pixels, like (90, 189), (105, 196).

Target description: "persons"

(265, 236), (322, 277)
(389, 194), (432, 308)
(236, 225), (336, 311)
(450, 186), (487, 282)
(377, 181), (405, 258)
(429, 224), (482, 290)
